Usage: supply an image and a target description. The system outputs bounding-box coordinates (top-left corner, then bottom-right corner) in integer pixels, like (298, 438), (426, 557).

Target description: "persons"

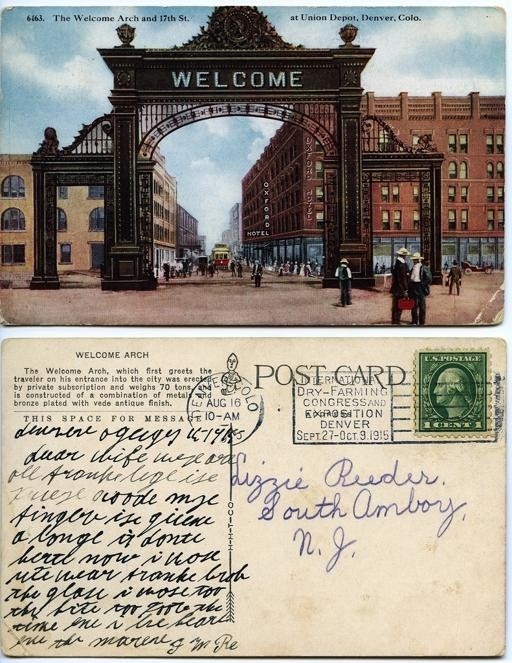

(431, 366), (474, 419)
(334, 256), (354, 306)
(382, 262), (386, 274)
(440, 262), (450, 287)
(374, 261), (379, 273)
(387, 246), (410, 323)
(446, 259), (462, 295)
(160, 246), (322, 287)
(407, 249), (432, 325)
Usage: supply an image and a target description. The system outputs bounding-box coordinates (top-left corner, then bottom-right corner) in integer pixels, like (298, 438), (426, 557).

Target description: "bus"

(212, 247), (230, 269)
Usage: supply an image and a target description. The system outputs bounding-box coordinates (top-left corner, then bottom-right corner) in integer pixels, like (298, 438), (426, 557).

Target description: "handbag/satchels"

(445, 281), (449, 286)
(398, 297), (416, 310)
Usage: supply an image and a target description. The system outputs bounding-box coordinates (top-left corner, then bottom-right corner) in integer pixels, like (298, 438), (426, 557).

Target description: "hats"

(410, 252), (424, 260)
(340, 258), (349, 264)
(396, 248), (411, 256)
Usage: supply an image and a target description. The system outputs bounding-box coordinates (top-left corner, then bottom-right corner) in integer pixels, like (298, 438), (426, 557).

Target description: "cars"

(461, 259), (493, 274)
